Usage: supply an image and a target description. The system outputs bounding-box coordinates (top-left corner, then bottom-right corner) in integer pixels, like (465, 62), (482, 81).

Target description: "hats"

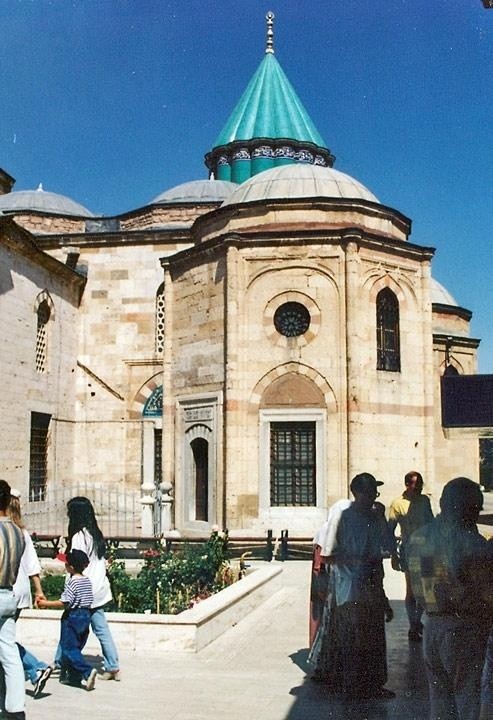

(350, 473), (382, 490)
(57, 549), (90, 570)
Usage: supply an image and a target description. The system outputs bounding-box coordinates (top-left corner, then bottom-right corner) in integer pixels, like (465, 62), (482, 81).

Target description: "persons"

(50, 496), (121, 682)
(36, 548), (98, 691)
(0, 479), (26, 720)
(306, 470), (493, 719)
(5, 489), (54, 698)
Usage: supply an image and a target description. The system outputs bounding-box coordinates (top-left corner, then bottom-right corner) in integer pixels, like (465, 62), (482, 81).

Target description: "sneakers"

(0, 711), (26, 720)
(50, 668), (66, 679)
(102, 670), (120, 681)
(61, 679), (82, 687)
(86, 669), (97, 691)
(33, 666), (53, 696)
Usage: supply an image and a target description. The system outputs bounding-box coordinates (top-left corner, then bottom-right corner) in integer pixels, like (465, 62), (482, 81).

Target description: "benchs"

(65, 537), (164, 559)
(29, 535), (61, 559)
